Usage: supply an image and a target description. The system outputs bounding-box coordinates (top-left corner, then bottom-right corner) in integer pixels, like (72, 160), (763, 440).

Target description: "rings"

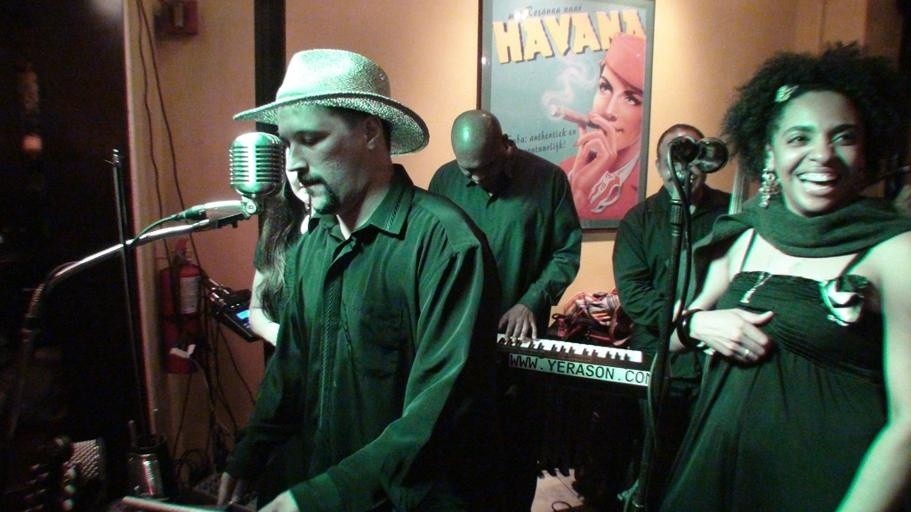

(743, 349), (750, 358)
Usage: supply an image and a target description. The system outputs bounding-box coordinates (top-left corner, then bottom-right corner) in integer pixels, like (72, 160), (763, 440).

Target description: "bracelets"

(677, 309), (708, 351)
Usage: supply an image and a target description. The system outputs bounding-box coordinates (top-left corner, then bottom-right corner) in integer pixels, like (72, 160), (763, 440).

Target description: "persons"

(247, 146), (316, 350)
(670, 49), (911, 512)
(560, 33), (645, 219)
(217, 49), (501, 512)
(612, 125), (732, 512)
(427, 110), (582, 343)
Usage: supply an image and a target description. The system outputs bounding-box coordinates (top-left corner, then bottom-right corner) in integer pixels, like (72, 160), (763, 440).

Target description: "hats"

(604, 32), (646, 94)
(232, 48), (430, 155)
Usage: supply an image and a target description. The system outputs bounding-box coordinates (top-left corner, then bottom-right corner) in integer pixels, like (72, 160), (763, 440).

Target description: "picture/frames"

(475, 0), (655, 234)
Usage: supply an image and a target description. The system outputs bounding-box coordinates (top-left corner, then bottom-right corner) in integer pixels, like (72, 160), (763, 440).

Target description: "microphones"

(669, 137), (728, 173)
(228, 131), (286, 214)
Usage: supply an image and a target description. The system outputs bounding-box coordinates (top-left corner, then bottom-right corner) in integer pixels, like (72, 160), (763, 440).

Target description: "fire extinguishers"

(159, 237), (210, 374)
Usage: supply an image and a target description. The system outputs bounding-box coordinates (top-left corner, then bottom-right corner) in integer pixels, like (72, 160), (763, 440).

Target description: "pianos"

(495, 328), (650, 391)
